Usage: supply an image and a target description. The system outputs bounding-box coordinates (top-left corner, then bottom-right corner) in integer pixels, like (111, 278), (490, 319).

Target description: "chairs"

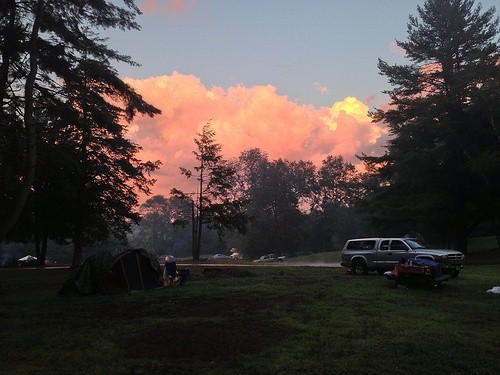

(164, 255), (191, 288)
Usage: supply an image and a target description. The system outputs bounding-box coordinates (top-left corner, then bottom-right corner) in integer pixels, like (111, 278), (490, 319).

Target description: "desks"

(393, 265), (425, 279)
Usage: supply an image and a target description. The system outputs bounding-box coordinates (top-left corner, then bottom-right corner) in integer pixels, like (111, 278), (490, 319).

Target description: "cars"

(252, 253), (286, 264)
(212, 252), (242, 261)
(16, 254), (38, 266)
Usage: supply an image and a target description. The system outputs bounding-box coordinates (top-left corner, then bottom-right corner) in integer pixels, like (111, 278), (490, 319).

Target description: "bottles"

(407, 259), (413, 266)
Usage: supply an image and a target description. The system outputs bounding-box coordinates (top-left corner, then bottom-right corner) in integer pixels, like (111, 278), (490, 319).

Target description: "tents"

(73, 248), (163, 294)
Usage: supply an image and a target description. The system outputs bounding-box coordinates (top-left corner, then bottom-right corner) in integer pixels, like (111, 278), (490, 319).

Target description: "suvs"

(339, 237), (465, 276)
